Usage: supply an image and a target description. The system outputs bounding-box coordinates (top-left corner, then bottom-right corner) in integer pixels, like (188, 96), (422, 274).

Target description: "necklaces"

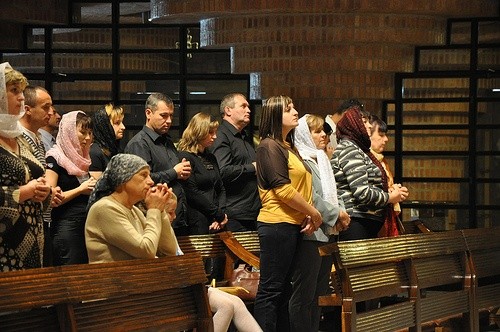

(111, 193), (121, 203)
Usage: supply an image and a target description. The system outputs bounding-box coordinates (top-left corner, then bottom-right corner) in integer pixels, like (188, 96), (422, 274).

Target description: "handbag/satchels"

(210, 265), (260, 297)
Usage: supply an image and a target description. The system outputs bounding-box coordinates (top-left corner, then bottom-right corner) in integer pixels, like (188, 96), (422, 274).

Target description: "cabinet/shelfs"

(0, 0), (261, 153)
(381, 17), (500, 231)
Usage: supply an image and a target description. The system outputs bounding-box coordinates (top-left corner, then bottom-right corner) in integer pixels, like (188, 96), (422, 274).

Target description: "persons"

(255, 94), (323, 332)
(0, 62), (410, 332)
(86, 153), (176, 303)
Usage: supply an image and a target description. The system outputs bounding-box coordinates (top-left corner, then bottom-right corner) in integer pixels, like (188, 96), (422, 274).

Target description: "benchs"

(0, 251), (214, 332)
(177, 230), (260, 316)
(317, 227), (500, 331)
